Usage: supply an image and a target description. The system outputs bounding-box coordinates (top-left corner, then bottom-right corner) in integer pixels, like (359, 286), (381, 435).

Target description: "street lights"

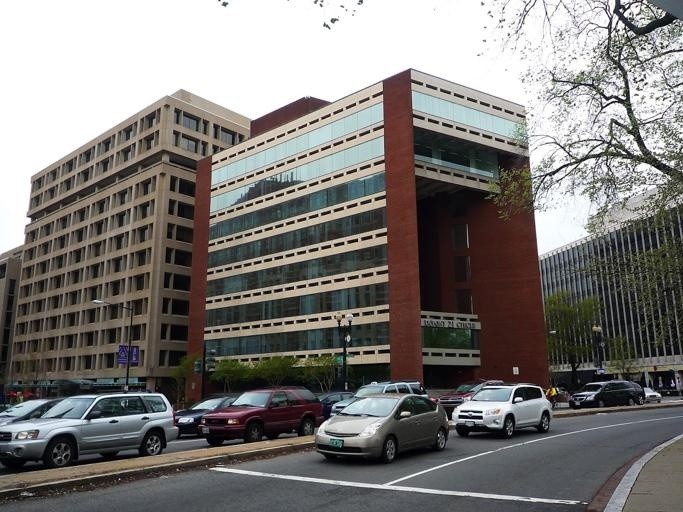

(335, 312), (356, 373)
(91, 300), (135, 388)
(590, 325), (604, 368)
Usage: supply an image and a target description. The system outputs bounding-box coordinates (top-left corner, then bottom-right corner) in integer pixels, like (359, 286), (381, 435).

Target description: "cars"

(313, 387), (353, 413)
(175, 391), (234, 436)
(0, 396), (65, 427)
(314, 393), (448, 463)
(567, 380), (662, 409)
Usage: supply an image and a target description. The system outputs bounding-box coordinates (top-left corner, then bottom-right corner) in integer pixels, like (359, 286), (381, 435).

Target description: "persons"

(545, 385), (558, 403)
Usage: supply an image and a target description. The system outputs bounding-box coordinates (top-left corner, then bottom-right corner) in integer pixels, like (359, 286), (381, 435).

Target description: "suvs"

(434, 378), (504, 410)
(199, 385), (322, 445)
(329, 379), (426, 415)
(450, 383), (552, 438)
(0, 390), (179, 464)
